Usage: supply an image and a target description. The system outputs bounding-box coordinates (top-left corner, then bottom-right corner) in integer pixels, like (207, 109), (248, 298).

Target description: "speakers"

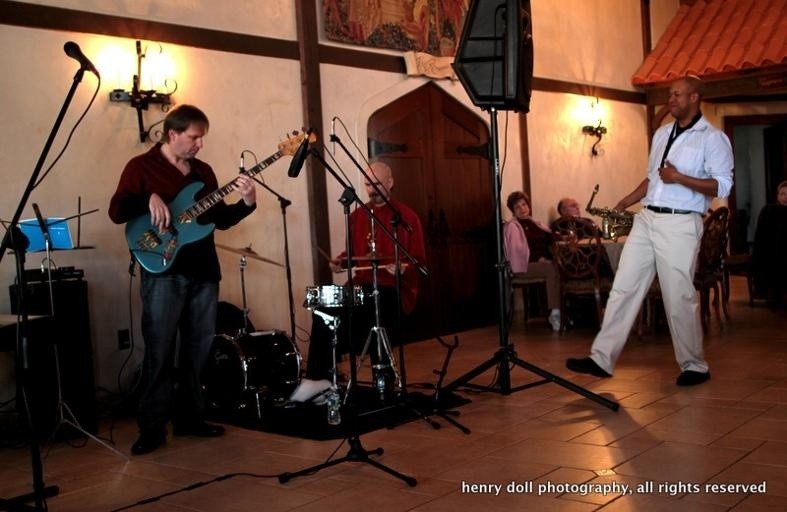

(450, 1), (535, 115)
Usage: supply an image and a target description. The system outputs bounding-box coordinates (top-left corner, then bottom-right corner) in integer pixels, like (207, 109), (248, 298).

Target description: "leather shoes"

(676, 371), (711, 386)
(131, 432), (166, 455)
(566, 358), (612, 377)
(173, 423), (225, 437)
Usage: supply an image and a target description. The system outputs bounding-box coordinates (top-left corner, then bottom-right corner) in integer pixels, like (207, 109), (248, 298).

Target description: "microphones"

(331, 119), (337, 154)
(64, 42), (99, 79)
(288, 136), (314, 177)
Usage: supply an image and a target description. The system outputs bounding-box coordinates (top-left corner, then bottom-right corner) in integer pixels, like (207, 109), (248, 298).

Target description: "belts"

(644, 205), (692, 214)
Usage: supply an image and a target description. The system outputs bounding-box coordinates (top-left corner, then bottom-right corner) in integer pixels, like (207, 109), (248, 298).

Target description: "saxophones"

(585, 184), (635, 241)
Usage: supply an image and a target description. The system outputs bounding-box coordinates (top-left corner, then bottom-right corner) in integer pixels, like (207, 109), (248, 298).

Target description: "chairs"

(502, 205), (752, 332)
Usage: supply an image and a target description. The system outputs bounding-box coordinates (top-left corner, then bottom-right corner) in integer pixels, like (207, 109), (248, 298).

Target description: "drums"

(308, 286), (367, 321)
(205, 330), (302, 413)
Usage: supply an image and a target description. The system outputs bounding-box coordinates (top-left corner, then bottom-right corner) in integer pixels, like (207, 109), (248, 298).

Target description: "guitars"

(125, 126), (317, 274)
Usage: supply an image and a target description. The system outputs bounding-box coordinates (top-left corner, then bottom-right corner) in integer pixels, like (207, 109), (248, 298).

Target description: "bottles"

(326, 386), (342, 425)
(602, 207), (611, 239)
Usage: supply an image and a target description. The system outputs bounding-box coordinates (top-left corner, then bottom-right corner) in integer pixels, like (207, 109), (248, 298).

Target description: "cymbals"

(345, 256), (383, 262)
(214, 242), (284, 270)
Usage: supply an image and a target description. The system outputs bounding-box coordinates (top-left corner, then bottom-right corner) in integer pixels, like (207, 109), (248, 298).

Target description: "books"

(17, 216), (75, 254)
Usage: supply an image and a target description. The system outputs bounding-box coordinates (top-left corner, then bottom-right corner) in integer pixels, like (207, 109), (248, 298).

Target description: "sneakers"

(289, 378), (332, 402)
(548, 315), (566, 331)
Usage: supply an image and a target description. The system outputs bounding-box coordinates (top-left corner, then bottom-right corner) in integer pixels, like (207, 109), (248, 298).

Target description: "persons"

(565, 75), (733, 386)
(108, 105), (257, 456)
(752, 180), (787, 310)
(291, 161), (424, 403)
(502, 191), (575, 332)
(549, 197), (614, 279)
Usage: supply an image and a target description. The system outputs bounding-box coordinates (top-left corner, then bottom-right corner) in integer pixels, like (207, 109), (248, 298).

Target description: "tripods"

(41, 240), (134, 465)
(278, 202), (420, 487)
(342, 213), (402, 406)
(443, 118), (621, 412)
